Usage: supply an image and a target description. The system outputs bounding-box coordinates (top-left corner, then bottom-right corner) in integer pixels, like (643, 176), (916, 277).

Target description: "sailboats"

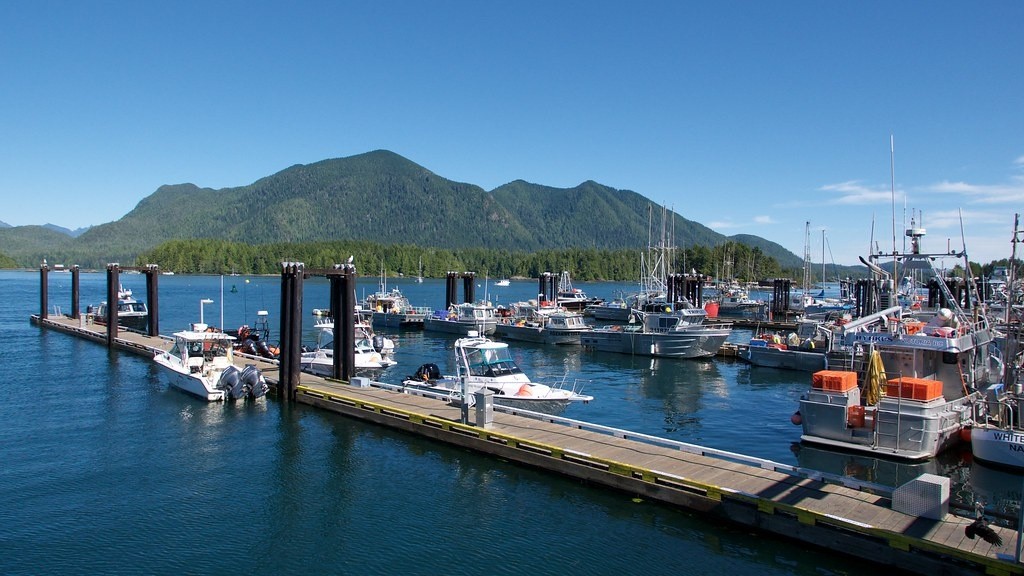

(970, 213), (1024, 474)
(703, 222), (949, 318)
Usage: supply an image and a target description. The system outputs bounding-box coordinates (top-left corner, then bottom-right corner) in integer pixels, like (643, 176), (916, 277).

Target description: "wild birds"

(964, 516), (1003, 548)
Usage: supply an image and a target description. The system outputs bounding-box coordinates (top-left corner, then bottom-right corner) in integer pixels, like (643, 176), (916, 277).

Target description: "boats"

(313, 321), (377, 328)
(354, 260), (437, 330)
(736, 255), (895, 373)
(422, 303), (497, 334)
(153, 330), (267, 403)
(400, 335), (594, 415)
(581, 200), (734, 361)
(509, 270), (605, 313)
(301, 328), (397, 382)
(493, 304), (591, 344)
(217, 327), (280, 360)
(795, 206), (998, 486)
(86, 283), (148, 330)
(414, 255), (423, 283)
